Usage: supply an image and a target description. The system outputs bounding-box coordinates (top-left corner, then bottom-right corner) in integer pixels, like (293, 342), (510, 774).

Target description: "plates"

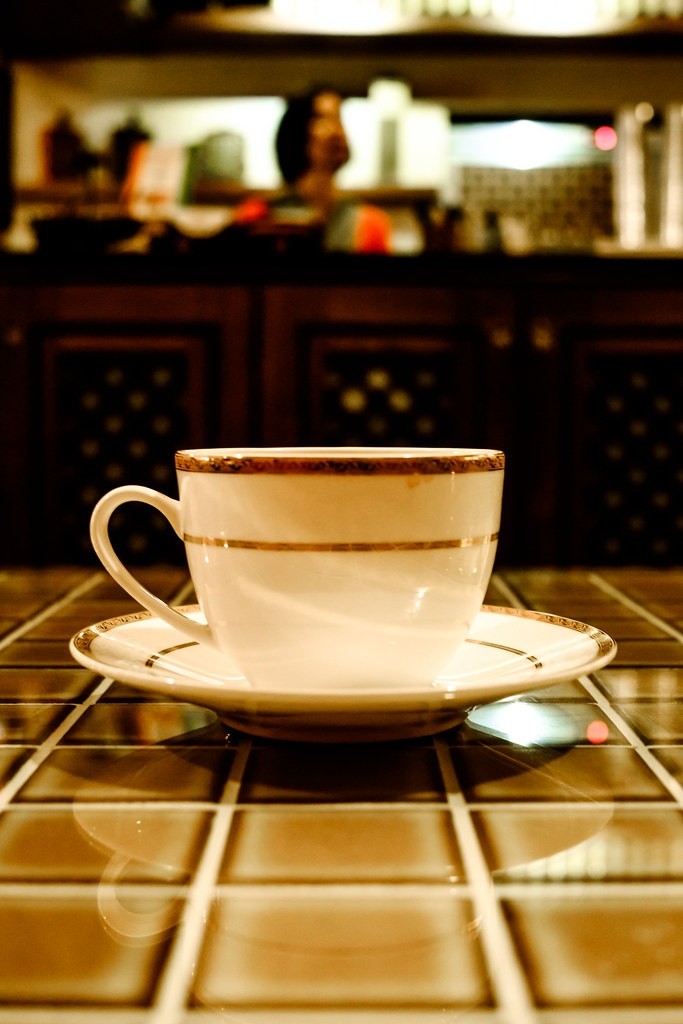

(69, 605), (618, 741)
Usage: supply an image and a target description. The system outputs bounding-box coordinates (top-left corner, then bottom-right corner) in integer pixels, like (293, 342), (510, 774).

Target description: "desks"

(0, 567), (683, 1024)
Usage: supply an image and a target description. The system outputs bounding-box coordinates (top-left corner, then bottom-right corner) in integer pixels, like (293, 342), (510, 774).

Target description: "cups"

(89, 448), (505, 685)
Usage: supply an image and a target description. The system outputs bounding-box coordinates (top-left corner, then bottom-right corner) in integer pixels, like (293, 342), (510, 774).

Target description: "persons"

(232, 87), (389, 251)
